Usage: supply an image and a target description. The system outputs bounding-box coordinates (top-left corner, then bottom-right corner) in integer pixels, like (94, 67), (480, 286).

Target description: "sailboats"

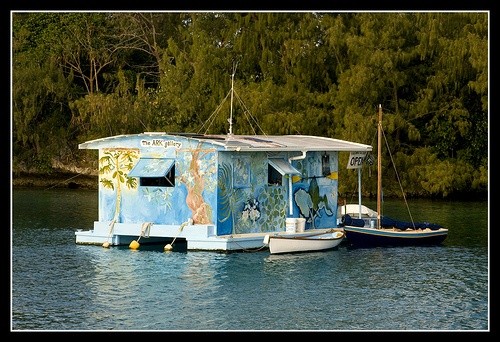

(342, 103), (450, 249)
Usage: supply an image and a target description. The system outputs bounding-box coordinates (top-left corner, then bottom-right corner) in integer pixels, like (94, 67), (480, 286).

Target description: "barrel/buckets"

(285, 217), (306, 233)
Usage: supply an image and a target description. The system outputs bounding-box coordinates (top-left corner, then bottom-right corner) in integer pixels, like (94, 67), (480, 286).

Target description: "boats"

(264, 227), (346, 256)
